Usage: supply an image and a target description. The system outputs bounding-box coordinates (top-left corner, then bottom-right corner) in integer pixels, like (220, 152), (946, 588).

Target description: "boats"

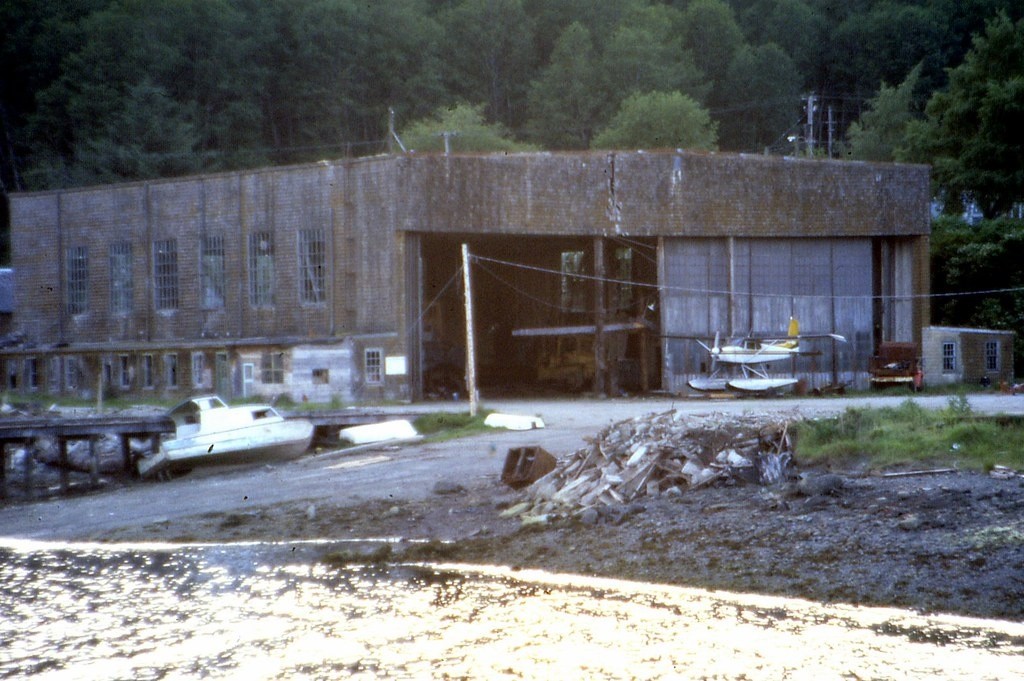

(727, 377), (799, 394)
(128, 392), (315, 481)
(687, 376), (733, 391)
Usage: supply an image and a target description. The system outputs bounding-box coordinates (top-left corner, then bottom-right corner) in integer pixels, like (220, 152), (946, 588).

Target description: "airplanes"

(693, 317), (850, 372)
